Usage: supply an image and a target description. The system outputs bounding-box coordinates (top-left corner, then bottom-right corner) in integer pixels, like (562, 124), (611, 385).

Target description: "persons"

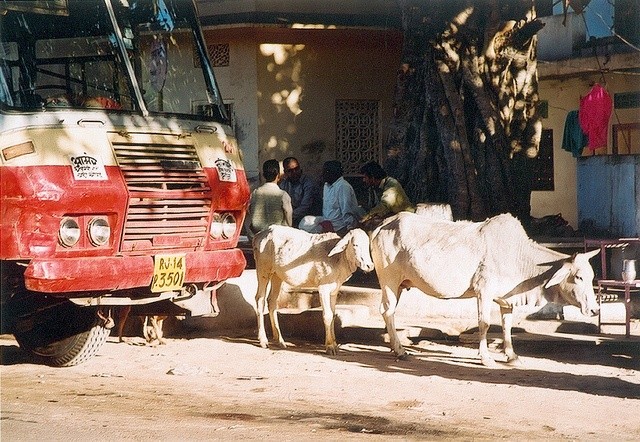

(297, 159), (359, 238)
(357, 160), (417, 233)
(277, 156), (316, 229)
(240, 157), (294, 247)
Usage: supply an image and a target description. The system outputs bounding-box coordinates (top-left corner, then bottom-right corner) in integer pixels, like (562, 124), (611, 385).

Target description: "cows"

(369, 210), (603, 367)
(251, 224), (373, 356)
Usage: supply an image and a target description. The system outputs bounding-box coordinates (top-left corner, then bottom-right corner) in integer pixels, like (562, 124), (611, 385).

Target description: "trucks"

(0, 1), (252, 367)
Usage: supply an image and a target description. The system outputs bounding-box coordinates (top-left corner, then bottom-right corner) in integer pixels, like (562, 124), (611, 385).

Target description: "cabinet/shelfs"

(583, 237), (640, 290)
(596, 277), (639, 339)
